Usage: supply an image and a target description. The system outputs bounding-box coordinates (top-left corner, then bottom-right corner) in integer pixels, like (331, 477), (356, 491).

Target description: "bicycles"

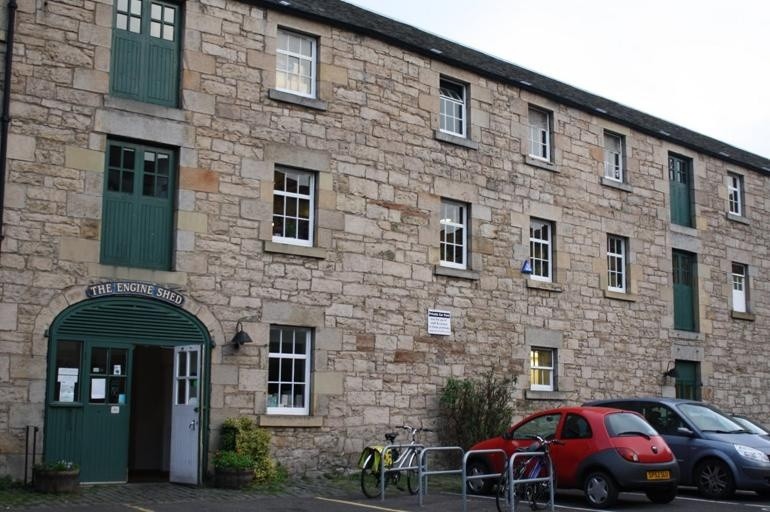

(495, 430), (565, 511)
(358, 422), (437, 499)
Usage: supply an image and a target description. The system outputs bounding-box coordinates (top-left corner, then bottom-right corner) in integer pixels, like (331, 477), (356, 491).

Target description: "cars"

(466, 404), (680, 506)
(729, 413), (768, 440)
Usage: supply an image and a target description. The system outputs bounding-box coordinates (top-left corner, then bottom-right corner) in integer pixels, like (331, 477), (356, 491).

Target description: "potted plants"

(237, 450), (258, 487)
(32, 462), (50, 493)
(49, 456), (81, 495)
(211, 449), (239, 488)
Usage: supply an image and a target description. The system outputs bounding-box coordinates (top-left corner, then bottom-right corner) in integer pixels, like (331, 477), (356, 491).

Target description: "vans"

(581, 399), (769, 498)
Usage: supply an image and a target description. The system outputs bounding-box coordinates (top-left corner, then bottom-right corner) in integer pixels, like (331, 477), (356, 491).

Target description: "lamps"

(231, 320), (253, 351)
